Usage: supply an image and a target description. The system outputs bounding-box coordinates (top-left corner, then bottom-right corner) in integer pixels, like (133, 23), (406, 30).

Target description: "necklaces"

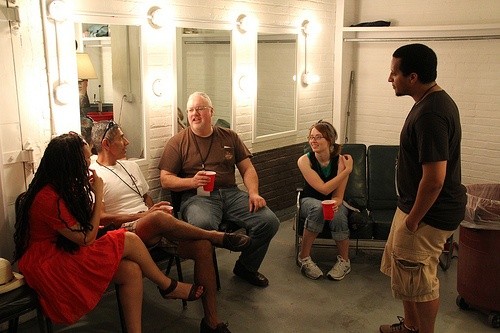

(96, 159), (143, 196)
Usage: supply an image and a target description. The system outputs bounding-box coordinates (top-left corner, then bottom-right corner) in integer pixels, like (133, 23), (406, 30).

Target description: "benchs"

(293, 143), (399, 255)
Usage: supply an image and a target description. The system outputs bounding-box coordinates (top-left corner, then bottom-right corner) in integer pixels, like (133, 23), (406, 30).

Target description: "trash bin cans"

(455, 183), (500, 328)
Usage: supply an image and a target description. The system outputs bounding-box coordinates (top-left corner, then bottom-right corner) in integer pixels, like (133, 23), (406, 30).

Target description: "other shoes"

(200, 318), (232, 333)
(214, 230), (251, 254)
(233, 265), (269, 288)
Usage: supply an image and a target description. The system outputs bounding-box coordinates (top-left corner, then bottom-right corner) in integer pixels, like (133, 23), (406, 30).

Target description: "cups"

(322, 200), (336, 220)
(202, 171), (216, 191)
(160, 205), (173, 215)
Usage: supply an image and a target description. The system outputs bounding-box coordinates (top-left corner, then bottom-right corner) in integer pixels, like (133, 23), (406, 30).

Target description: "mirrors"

(70, 10), (150, 168)
(172, 24), (235, 138)
(252, 32), (297, 145)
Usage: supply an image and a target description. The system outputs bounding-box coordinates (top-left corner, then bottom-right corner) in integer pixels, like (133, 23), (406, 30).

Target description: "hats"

(0, 258), (25, 295)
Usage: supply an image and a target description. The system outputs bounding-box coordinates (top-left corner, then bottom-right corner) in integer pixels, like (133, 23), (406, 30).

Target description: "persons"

(14, 131), (207, 333)
(297, 119), (354, 282)
(90, 122), (254, 333)
(378, 44), (469, 333)
(156, 90), (279, 288)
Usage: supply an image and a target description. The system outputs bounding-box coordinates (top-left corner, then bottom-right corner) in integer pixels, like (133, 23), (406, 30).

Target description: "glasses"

(305, 136), (325, 141)
(184, 106), (213, 113)
(100, 120), (118, 144)
(69, 131), (87, 148)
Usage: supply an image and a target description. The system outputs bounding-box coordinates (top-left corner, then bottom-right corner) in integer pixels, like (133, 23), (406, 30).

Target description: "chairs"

(15, 188), (248, 332)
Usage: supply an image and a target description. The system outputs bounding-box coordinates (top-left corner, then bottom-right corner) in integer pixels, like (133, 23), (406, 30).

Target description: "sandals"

(157, 277), (204, 302)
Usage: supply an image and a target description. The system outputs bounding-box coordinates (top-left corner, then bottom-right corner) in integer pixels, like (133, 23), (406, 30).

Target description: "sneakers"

(379, 316), (420, 333)
(326, 255), (352, 281)
(296, 252), (324, 280)
